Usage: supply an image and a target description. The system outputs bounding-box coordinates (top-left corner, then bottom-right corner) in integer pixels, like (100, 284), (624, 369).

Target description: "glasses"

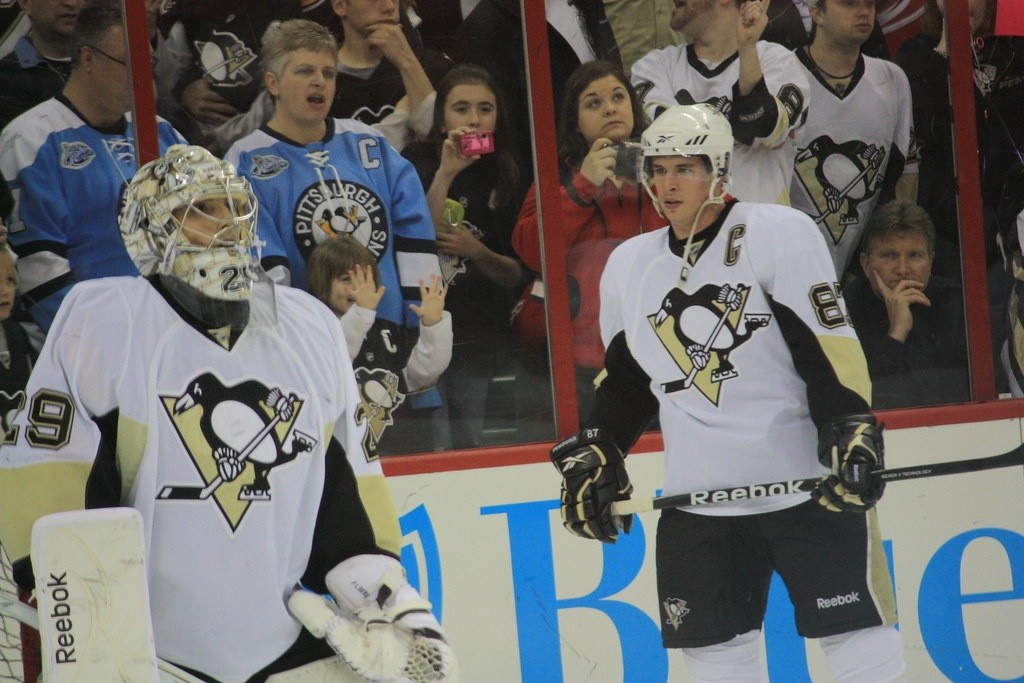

(82, 44), (159, 69)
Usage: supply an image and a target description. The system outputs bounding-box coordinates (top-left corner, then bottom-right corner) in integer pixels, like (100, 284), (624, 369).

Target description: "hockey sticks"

(0, 600), (222, 683)
(609, 443), (1024, 514)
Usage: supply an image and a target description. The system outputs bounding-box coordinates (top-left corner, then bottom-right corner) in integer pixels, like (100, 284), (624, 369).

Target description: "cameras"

(603, 139), (640, 177)
(461, 131), (494, 155)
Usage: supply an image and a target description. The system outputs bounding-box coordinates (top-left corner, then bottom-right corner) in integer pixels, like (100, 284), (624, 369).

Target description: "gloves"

(324, 554), (450, 683)
(550, 428), (634, 544)
(811, 414), (886, 513)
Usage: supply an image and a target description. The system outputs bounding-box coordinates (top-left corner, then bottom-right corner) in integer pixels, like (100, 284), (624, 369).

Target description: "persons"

(511, 0), (1024, 444)
(0, 0), (624, 455)
(0, 144), (454, 683)
(550, 102), (906, 683)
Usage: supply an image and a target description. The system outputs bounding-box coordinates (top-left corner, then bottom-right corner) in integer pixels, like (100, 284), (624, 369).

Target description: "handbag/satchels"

(508, 277), (548, 352)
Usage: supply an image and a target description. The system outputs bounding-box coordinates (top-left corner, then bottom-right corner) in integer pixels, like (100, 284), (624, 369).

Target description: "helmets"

(641, 104), (735, 177)
(118, 145), (254, 301)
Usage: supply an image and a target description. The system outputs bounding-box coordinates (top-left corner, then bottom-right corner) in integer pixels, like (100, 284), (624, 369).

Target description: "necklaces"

(808, 45), (862, 79)
